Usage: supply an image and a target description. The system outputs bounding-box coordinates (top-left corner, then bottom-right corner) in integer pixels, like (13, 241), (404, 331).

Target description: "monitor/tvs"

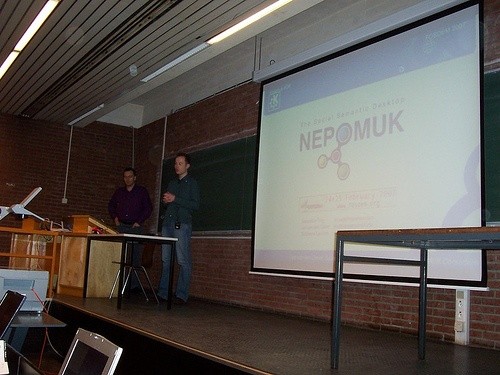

(58, 327), (123, 375)
(0, 290), (27, 339)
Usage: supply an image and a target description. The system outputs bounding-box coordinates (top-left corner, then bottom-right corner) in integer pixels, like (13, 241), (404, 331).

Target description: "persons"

(153, 152), (201, 307)
(107, 166), (152, 296)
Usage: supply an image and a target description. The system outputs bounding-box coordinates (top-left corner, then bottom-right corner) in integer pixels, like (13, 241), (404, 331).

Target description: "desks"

(82, 234), (178, 311)
(331, 228), (500, 369)
(7, 311), (67, 357)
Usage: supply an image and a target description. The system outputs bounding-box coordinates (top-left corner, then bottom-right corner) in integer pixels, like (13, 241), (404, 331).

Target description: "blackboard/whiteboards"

(156, 134), (258, 239)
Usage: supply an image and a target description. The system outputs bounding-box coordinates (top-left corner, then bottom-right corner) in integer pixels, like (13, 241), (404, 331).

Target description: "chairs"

(108, 242), (160, 304)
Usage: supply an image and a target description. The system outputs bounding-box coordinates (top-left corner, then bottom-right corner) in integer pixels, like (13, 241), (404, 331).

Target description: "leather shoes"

(124, 288), (137, 296)
(155, 296), (185, 306)
